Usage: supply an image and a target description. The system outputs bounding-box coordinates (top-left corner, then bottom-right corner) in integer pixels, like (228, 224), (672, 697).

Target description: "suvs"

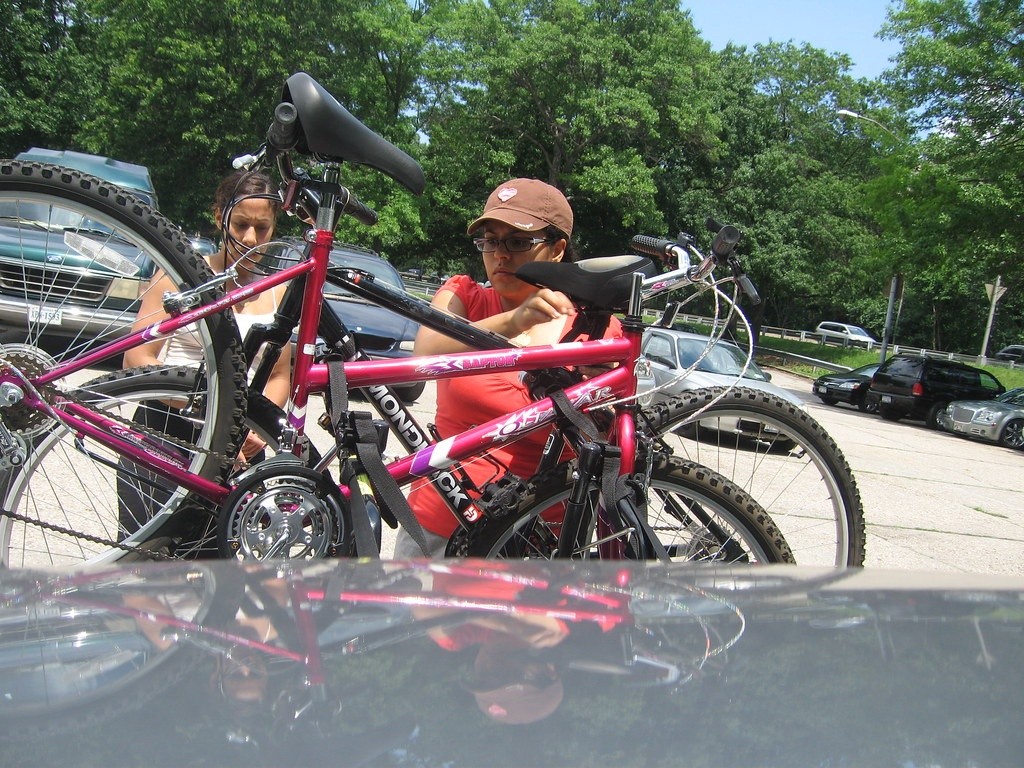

(275, 232), (431, 403)
(0, 147), (161, 366)
(865, 351), (1009, 432)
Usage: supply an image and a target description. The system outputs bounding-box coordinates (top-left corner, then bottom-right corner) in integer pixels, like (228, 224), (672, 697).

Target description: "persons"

(116, 170), (291, 563)
(395, 178), (632, 561)
(106, 563), (638, 746)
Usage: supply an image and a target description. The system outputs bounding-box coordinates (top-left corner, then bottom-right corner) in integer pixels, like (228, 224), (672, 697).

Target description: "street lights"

(838, 110), (919, 345)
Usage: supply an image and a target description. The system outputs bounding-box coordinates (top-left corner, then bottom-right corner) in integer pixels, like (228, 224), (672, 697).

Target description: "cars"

(996, 344), (1024, 365)
(184, 234), (223, 259)
(640, 326), (807, 454)
(409, 267), (492, 289)
(811, 360), (884, 406)
(937, 386), (1024, 451)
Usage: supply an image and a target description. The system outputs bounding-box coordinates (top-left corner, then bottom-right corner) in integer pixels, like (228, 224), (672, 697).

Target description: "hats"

(472, 677), (563, 725)
(467, 179), (573, 240)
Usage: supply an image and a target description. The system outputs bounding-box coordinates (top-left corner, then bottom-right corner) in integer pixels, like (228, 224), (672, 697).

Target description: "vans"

(815, 321), (876, 349)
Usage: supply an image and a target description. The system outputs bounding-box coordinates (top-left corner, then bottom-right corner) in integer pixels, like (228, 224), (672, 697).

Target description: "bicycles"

(0, 69), (874, 582)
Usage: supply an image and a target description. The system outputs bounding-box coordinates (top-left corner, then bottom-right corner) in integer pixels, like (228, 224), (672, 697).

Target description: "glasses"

(473, 237), (555, 253)
